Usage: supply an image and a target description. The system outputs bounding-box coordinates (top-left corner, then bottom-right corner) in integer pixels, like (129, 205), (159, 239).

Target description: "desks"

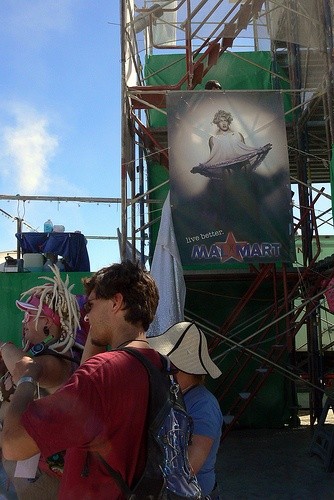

(15, 232), (90, 272)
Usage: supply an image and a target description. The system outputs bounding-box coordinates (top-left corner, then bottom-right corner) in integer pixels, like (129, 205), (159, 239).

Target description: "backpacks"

(100, 346), (203, 499)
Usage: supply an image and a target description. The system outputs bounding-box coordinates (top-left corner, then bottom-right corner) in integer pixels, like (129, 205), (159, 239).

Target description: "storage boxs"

(23, 253), (43, 272)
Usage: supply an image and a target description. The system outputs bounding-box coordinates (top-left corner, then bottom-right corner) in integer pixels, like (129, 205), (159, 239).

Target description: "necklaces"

(115, 339), (149, 349)
(181, 383), (194, 393)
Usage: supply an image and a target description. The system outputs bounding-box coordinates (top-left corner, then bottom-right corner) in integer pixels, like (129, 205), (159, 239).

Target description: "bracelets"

(0, 342), (8, 351)
(17, 377), (36, 387)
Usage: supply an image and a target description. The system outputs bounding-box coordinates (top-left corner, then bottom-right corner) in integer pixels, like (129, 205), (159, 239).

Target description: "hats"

(147, 321), (223, 380)
(15, 283), (91, 348)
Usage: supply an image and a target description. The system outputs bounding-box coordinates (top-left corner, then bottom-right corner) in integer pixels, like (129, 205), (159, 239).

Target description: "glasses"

(20, 311), (49, 324)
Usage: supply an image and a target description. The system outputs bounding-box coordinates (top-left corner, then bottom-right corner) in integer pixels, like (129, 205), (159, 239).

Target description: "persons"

(206, 80), (222, 90)
(190, 110), (273, 181)
(0, 264), (81, 500)
(146, 321), (222, 500)
(2, 259), (163, 500)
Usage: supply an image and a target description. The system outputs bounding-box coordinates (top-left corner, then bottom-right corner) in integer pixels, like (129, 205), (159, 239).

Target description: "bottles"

(26, 334), (55, 357)
(44, 219), (53, 232)
(56, 259), (65, 271)
(44, 258), (55, 272)
